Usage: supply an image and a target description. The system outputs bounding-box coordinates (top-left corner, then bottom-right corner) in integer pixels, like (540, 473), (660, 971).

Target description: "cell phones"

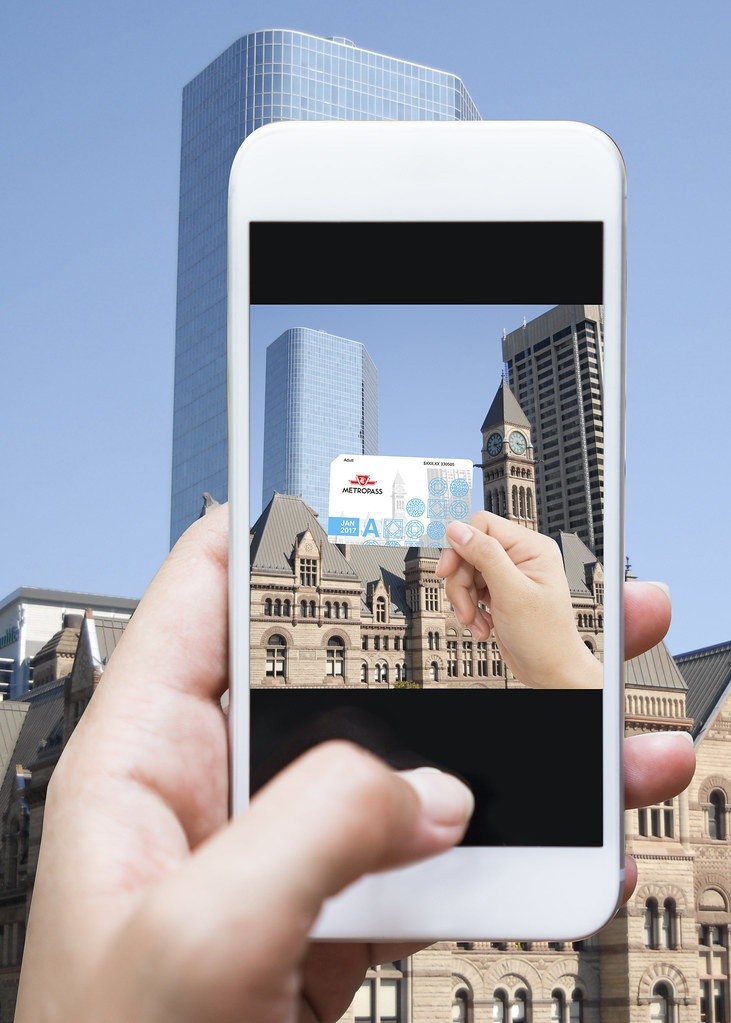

(226, 114), (621, 947)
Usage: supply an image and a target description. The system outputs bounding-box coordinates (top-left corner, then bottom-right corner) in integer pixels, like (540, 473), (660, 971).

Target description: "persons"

(0, 485), (698, 1023)
(426, 509), (605, 690)
